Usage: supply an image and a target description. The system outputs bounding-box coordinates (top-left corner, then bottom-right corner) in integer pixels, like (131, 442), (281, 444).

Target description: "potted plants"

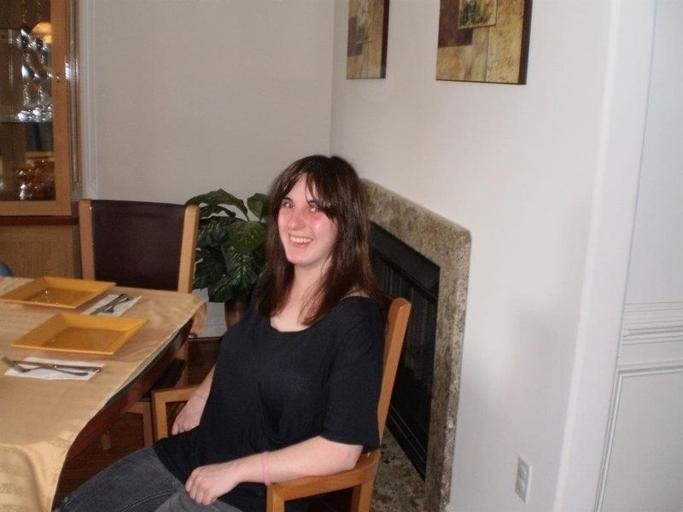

(184, 188), (268, 328)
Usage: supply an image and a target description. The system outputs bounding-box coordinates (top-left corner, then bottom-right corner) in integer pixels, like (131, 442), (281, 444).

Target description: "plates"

(1, 278), (112, 310)
(12, 311), (150, 358)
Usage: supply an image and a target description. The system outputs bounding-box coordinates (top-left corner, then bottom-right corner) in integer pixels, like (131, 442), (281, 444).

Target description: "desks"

(0, 275), (196, 464)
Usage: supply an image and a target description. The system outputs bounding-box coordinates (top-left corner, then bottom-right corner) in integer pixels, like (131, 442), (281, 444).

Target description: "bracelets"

(191, 392), (207, 402)
(263, 450), (271, 486)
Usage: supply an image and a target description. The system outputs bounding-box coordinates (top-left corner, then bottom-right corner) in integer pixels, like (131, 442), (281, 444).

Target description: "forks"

(104, 296), (137, 313)
(2, 354), (89, 379)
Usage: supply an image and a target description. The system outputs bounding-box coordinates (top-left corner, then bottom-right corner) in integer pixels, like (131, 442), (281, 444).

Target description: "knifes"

(11, 359), (104, 373)
(90, 292), (128, 315)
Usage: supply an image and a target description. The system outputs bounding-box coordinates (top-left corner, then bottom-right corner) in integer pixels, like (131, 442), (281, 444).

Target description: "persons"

(52, 153), (382, 512)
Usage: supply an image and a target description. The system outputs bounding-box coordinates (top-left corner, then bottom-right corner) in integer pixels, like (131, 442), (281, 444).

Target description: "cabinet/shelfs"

(0, 2), (74, 216)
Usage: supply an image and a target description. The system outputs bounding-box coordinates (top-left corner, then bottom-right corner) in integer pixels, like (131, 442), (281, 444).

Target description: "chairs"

(77, 198), (201, 448)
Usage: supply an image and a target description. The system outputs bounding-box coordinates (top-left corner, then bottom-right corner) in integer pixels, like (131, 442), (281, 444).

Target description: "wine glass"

(13, 0), (51, 121)
(17, 158), (54, 199)
